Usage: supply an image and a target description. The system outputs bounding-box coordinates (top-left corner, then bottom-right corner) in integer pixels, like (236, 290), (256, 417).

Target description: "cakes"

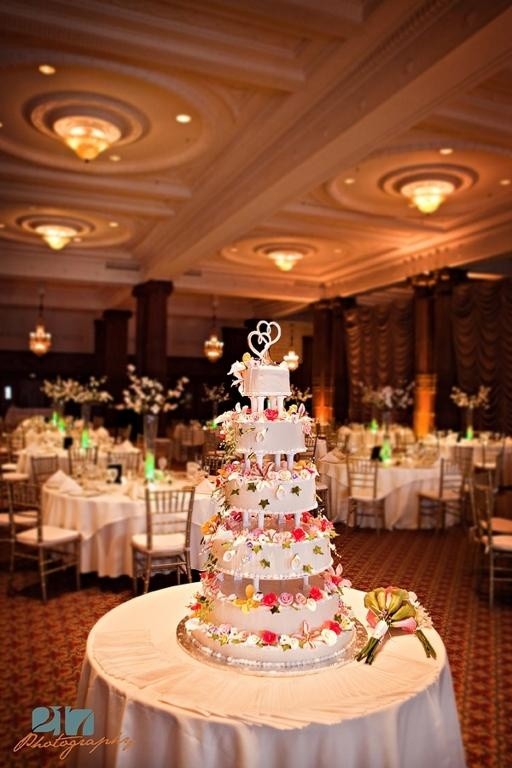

(187, 365), (354, 668)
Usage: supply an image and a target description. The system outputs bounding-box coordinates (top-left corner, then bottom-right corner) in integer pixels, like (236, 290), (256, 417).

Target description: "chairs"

(0, 413), (510, 611)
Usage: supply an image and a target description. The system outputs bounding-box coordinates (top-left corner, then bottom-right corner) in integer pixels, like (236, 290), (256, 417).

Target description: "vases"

(51, 398), (91, 449)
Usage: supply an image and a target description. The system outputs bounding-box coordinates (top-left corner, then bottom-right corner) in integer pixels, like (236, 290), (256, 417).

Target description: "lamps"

(27, 292), (51, 357)
(202, 293), (224, 362)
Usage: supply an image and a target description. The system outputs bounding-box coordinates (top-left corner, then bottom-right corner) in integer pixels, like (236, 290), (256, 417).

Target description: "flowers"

(450, 383), (491, 412)
(362, 384), (414, 410)
(36, 370), (189, 415)
(355, 586), (432, 665)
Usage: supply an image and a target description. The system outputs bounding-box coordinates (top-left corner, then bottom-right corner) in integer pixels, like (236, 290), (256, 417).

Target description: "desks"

(74, 581), (464, 766)
